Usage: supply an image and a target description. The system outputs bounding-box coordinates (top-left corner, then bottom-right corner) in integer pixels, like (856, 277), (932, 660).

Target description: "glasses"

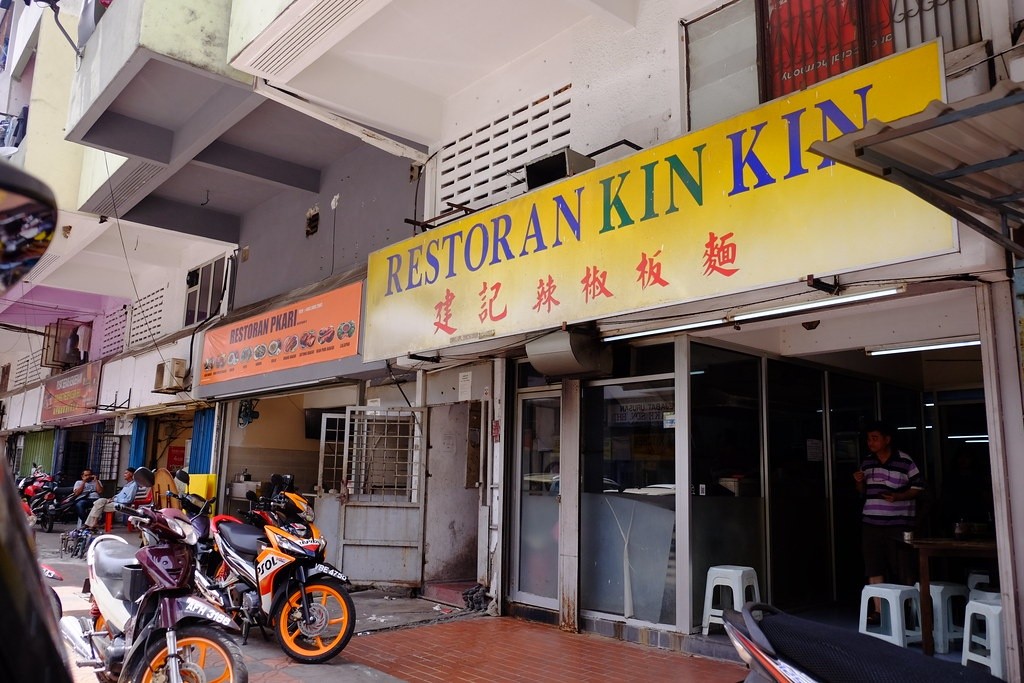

(872, 465), (891, 482)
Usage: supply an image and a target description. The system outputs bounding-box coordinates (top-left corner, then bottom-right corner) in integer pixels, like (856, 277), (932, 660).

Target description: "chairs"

(104, 485), (154, 533)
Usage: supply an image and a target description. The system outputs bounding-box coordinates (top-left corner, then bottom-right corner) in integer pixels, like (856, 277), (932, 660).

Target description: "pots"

(234, 472), (252, 482)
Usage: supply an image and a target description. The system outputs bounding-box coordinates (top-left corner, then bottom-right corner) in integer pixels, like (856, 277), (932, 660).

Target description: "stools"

(858, 583), (922, 648)
(912, 581), (968, 654)
(961, 599), (1006, 681)
(702, 565), (763, 636)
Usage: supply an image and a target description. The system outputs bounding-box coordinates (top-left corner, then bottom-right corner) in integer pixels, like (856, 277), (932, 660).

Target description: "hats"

(127, 467), (136, 473)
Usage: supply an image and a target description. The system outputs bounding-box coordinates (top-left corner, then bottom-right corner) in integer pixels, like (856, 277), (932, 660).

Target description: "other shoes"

(866, 610), (881, 626)
(86, 526), (96, 530)
(79, 524), (89, 529)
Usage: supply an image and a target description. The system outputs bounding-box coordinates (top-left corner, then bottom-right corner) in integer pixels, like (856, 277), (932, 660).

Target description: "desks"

(227, 497), (256, 517)
(888, 534), (997, 657)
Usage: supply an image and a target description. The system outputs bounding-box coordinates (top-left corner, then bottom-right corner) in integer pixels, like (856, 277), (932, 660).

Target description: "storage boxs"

(229, 481), (271, 499)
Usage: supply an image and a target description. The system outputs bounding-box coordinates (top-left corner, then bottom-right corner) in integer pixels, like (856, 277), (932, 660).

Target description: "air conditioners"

(153, 358), (187, 391)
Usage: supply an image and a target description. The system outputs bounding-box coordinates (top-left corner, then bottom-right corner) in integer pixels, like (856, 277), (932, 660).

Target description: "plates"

(227, 341), (280, 366)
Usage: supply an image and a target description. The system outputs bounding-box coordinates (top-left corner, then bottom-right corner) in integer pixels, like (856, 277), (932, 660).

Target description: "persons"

(854, 421), (923, 629)
(80, 468), (138, 533)
(73, 469), (103, 525)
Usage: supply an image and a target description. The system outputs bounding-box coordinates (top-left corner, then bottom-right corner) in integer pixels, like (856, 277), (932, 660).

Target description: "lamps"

(601, 310), (729, 342)
(729, 282), (908, 322)
(865, 335), (981, 355)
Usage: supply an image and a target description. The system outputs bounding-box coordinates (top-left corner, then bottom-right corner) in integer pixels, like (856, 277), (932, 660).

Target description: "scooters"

(56, 465), (249, 683)
(194, 473), (357, 664)
(139, 470), (217, 587)
(720, 601), (1009, 683)
(12, 461), (60, 534)
